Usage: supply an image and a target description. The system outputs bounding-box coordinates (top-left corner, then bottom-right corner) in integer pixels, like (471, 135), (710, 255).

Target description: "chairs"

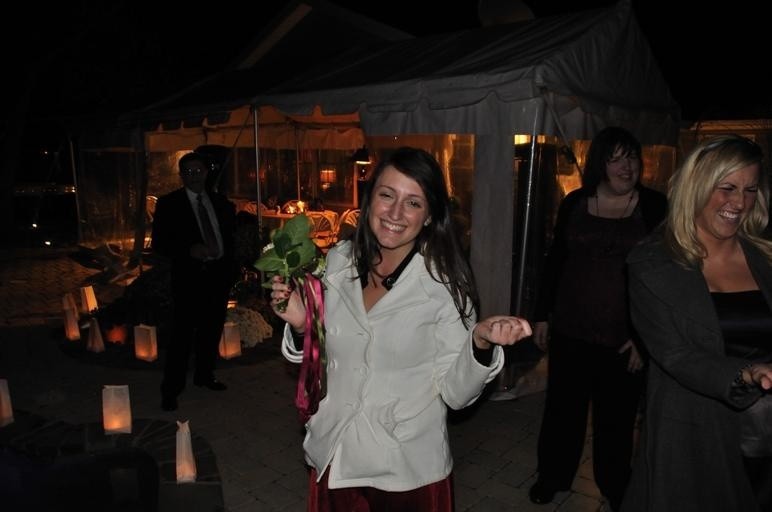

(144, 195), (159, 248)
(226, 192), (361, 251)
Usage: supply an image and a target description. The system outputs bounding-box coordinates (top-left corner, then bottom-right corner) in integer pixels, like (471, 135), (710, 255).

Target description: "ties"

(195, 194), (220, 258)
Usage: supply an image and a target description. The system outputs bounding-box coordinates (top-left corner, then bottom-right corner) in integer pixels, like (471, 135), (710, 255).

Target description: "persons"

(444, 196), (468, 250)
(620, 134), (772, 512)
(151, 153), (234, 411)
(269, 146), (532, 512)
(530, 125), (668, 512)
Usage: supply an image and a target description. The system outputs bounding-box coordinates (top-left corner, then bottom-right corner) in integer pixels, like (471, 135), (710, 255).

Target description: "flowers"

(252, 212), (327, 316)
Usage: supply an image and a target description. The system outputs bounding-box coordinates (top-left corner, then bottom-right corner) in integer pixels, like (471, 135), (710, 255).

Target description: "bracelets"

(733, 365), (754, 390)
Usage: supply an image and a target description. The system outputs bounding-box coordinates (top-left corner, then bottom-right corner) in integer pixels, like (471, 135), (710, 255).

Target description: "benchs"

(0, 409), (224, 512)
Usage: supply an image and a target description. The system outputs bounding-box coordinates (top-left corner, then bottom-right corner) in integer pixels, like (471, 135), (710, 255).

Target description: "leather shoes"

(160, 384), (179, 412)
(529, 481), (557, 505)
(193, 370), (228, 391)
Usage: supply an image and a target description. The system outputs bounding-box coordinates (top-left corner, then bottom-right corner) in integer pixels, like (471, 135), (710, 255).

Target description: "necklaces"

(595, 189), (637, 253)
